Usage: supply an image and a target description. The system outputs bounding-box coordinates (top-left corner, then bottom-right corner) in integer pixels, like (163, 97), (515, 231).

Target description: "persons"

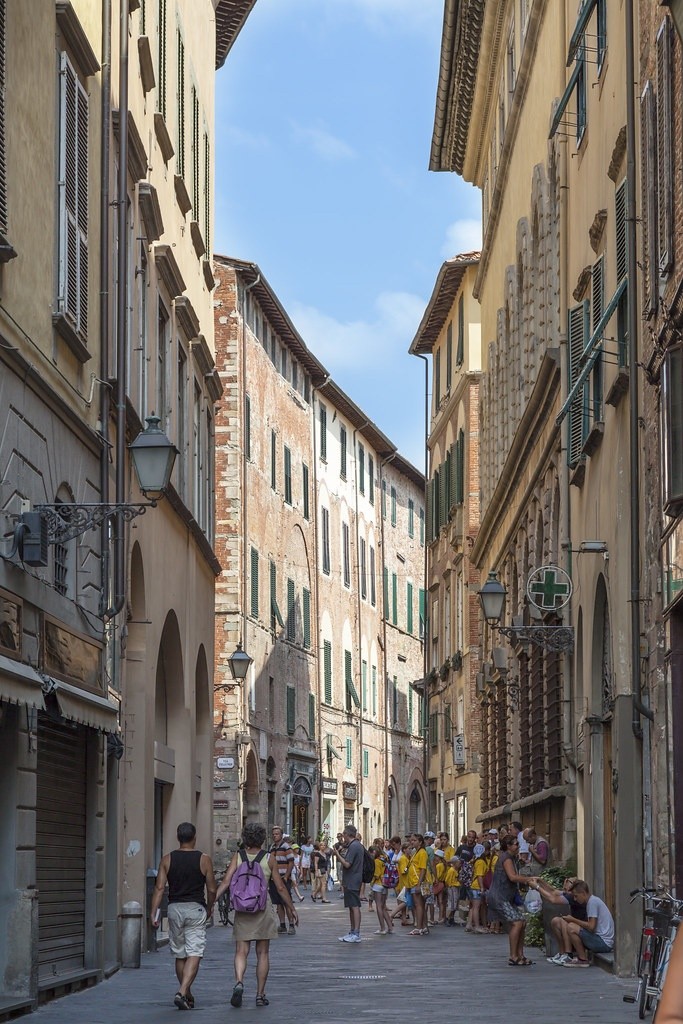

(527, 875), (614, 968)
(214, 823), (300, 1008)
(487, 834), (537, 966)
(281, 832), (335, 902)
(267, 823), (296, 936)
(368, 822), (551, 935)
(653, 904), (683, 1024)
(334, 824), (366, 944)
(150, 822), (216, 1010)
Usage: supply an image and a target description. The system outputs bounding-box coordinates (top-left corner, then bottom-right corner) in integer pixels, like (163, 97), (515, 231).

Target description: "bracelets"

(535, 885), (539, 890)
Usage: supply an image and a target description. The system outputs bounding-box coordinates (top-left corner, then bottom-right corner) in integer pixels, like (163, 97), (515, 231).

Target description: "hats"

(404, 829), (498, 860)
(291, 843), (300, 849)
(336, 833), (343, 838)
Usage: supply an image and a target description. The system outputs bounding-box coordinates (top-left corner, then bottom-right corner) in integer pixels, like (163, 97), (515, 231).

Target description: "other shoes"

(387, 928), (395, 933)
(289, 886), (374, 912)
(392, 910), (590, 967)
(278, 926), (287, 933)
(288, 927), (296, 935)
(374, 930), (386, 935)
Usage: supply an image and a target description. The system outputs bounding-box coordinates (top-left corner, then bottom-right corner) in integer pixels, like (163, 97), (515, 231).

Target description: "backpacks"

(378, 858), (399, 889)
(229, 849), (268, 913)
(458, 859), (481, 887)
(361, 845), (376, 883)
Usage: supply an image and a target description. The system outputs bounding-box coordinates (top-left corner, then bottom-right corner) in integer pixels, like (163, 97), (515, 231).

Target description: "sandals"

(174, 992), (191, 1010)
(407, 928), (424, 935)
(230, 981), (243, 1008)
(186, 993), (194, 1009)
(256, 994), (269, 1006)
(421, 928), (429, 934)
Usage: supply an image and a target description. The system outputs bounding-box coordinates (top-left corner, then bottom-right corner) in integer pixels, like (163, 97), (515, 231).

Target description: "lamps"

(213, 644), (254, 693)
(33, 416), (181, 548)
(477, 571), (575, 657)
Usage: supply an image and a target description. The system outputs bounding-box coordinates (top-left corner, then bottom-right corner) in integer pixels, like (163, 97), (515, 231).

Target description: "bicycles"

(623, 886), (683, 1022)
(212, 864), (234, 926)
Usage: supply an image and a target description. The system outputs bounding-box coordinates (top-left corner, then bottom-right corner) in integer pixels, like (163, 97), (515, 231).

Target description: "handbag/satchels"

(421, 882), (430, 898)
(326, 874), (334, 891)
(432, 880), (444, 894)
(483, 870), (493, 890)
(524, 886), (542, 914)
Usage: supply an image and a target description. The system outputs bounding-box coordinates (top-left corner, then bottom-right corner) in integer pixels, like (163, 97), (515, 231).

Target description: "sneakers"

(338, 934), (354, 941)
(343, 935), (361, 943)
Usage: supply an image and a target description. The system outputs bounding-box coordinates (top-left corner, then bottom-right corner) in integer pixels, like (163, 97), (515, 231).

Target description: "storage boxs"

(653, 908), (683, 938)
